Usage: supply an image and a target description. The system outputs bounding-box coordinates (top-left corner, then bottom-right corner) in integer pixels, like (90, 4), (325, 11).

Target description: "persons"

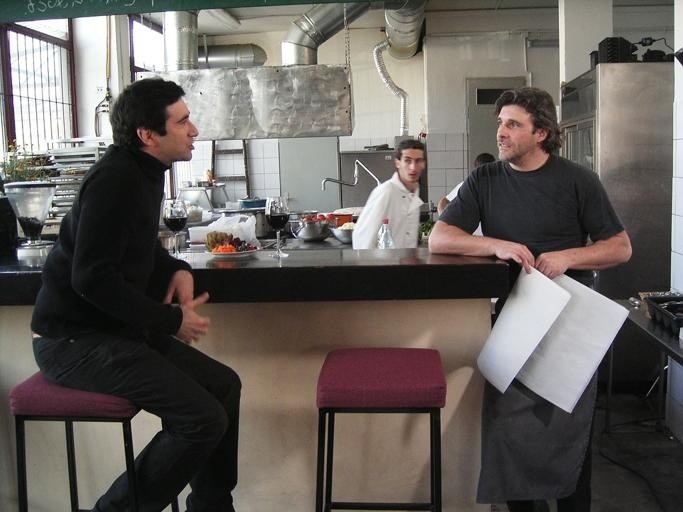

(29, 76), (243, 512)
(437, 153), (495, 238)
(428, 86), (632, 511)
(351, 139), (426, 250)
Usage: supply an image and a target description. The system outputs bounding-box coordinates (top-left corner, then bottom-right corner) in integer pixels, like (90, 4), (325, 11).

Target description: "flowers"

(0, 138), (56, 183)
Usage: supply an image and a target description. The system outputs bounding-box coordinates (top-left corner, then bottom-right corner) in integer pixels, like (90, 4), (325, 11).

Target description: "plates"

(205, 246), (260, 258)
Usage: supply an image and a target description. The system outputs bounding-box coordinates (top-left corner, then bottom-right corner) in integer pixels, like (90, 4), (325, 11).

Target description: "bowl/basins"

(328, 227), (353, 243)
(277, 211), (318, 233)
(288, 218), (337, 242)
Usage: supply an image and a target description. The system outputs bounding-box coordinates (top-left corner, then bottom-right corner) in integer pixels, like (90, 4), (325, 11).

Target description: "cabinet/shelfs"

(560, 62), (674, 393)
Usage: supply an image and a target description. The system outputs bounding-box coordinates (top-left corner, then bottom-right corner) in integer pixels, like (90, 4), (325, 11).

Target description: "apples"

(304, 214), (335, 222)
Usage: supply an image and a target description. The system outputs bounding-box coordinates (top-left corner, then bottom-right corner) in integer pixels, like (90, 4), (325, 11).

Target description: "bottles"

(377, 219), (394, 248)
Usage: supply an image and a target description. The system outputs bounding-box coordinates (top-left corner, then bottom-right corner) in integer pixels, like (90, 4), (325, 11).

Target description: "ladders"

(212, 140), (251, 208)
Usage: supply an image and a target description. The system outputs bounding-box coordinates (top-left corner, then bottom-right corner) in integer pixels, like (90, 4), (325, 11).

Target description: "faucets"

(320, 159), (380, 192)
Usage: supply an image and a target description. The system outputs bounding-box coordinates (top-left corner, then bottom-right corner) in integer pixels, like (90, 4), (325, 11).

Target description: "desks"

(605, 300), (683, 427)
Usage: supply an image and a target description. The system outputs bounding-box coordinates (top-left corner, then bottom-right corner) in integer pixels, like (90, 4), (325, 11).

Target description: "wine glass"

(265, 196), (289, 258)
(162, 200), (188, 260)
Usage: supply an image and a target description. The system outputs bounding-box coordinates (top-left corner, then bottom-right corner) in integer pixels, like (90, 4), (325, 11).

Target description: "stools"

(10, 371), (179, 512)
(315, 348), (447, 512)
